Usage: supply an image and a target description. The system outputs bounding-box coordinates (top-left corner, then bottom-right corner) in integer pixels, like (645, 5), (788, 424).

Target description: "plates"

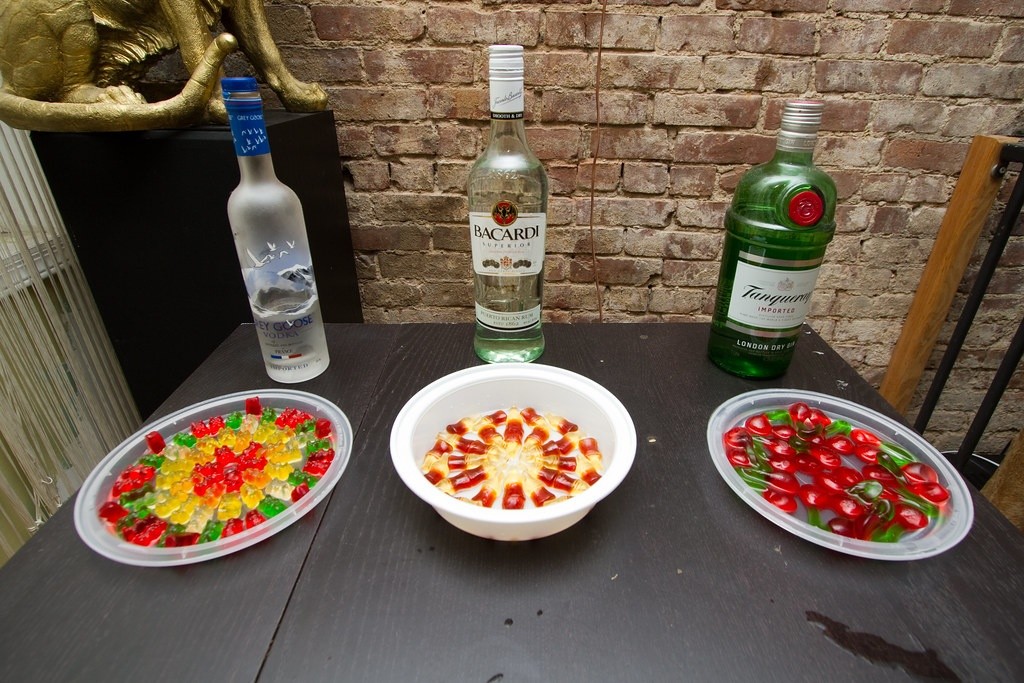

(707, 388), (974, 560)
(72, 388), (354, 567)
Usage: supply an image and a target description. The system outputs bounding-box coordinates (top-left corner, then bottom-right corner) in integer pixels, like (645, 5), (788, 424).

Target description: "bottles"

(468, 45), (549, 364)
(707, 102), (838, 379)
(219, 76), (330, 383)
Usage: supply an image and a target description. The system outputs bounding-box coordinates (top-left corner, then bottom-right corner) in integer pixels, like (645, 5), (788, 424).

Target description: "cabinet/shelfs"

(27, 104), (362, 423)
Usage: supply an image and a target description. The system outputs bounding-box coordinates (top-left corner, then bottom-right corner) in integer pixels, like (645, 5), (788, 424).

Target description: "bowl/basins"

(389, 363), (637, 540)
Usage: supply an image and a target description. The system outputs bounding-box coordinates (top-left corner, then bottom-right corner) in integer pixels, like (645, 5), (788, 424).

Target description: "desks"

(0, 312), (1024, 683)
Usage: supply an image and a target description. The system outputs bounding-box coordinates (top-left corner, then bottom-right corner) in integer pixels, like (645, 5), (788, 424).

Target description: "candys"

(420, 406), (603, 510)
(725, 402), (949, 545)
(98, 398), (335, 548)
